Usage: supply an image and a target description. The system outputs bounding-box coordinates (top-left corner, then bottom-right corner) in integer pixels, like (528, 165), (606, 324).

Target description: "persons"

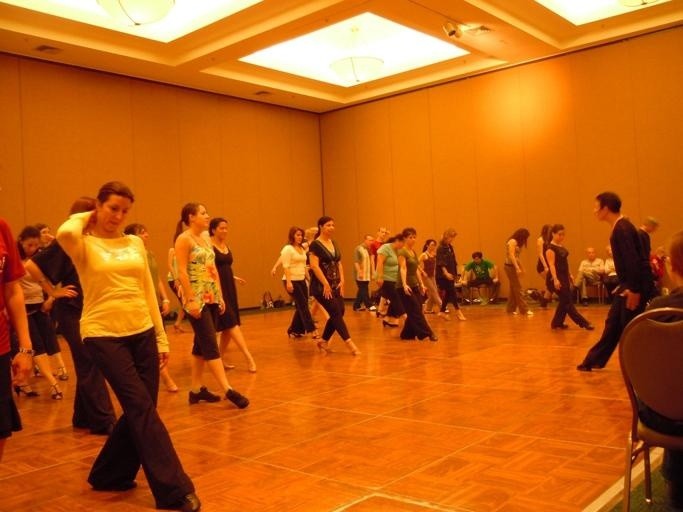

(272, 216), (467, 356)
(2, 181), (258, 510)
(459, 191), (673, 370)
(632, 230), (683, 508)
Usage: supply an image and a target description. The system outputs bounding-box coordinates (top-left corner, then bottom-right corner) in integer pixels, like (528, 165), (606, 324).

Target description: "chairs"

(471, 286), (492, 305)
(577, 284), (606, 306)
(619, 308), (683, 512)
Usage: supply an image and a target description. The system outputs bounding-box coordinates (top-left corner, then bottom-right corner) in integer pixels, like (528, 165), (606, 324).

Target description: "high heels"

(347, 343), (362, 357)
(172, 323), (187, 333)
(507, 309), (535, 316)
(35, 366), (67, 400)
(12, 383), (39, 398)
(309, 328), (336, 353)
(287, 328), (303, 338)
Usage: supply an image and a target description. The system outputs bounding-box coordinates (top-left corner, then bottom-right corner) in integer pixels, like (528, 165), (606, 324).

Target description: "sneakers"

(423, 308), (466, 321)
(224, 388), (249, 409)
(464, 298), (493, 305)
(188, 386), (221, 404)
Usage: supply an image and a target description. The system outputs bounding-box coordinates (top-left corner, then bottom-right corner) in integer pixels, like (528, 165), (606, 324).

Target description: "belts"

(504, 263), (513, 266)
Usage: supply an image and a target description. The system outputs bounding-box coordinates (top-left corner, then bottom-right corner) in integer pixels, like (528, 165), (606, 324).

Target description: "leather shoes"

(578, 356), (606, 371)
(429, 334), (437, 341)
(353, 306), (401, 328)
(221, 359), (235, 371)
(582, 298), (589, 305)
(570, 286), (579, 294)
(606, 297), (612, 304)
(164, 378), (179, 393)
(246, 356), (257, 374)
(551, 322), (595, 331)
